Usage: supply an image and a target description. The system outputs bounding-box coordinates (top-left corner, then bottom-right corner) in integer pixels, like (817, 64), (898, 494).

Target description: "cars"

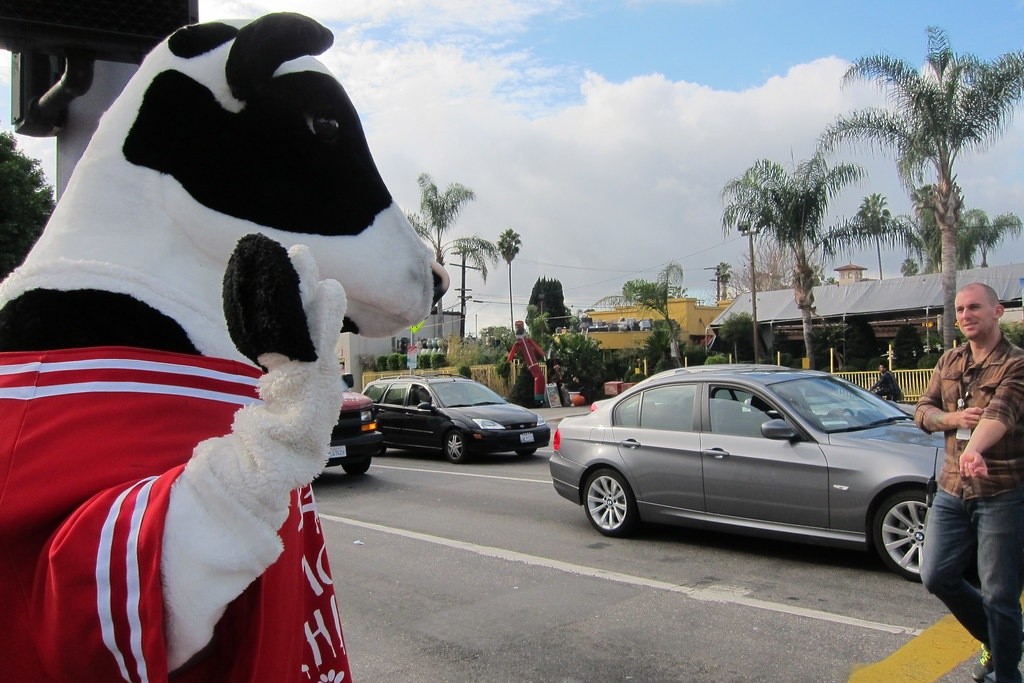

(549, 363), (946, 583)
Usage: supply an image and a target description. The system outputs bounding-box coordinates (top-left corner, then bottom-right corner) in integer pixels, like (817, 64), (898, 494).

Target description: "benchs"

(632, 395), (694, 432)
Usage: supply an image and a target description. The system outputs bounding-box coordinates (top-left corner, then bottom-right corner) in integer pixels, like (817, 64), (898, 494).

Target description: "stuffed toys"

(0, 11), (451, 683)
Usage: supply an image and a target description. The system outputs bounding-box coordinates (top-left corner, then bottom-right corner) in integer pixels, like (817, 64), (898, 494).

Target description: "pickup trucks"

(326, 372), (384, 476)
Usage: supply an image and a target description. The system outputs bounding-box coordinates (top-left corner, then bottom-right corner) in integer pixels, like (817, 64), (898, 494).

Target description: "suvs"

(362, 373), (549, 463)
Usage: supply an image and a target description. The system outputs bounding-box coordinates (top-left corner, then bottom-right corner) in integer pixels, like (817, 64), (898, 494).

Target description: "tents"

(704, 264), (1024, 365)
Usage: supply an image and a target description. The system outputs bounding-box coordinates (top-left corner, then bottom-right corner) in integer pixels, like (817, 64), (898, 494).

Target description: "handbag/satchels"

(895, 391), (905, 400)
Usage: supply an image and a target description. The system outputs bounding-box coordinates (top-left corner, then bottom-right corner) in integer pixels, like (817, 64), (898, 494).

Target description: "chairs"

(749, 394), (785, 438)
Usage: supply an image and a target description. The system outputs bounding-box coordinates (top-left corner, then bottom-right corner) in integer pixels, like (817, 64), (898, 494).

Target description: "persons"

(914, 282), (1024, 683)
(867, 361), (901, 402)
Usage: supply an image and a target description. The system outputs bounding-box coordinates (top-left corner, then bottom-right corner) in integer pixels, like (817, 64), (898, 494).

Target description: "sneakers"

(971, 644), (993, 682)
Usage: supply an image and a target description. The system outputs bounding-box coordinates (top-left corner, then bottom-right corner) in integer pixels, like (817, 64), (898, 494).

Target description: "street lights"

(737, 219), (760, 363)
